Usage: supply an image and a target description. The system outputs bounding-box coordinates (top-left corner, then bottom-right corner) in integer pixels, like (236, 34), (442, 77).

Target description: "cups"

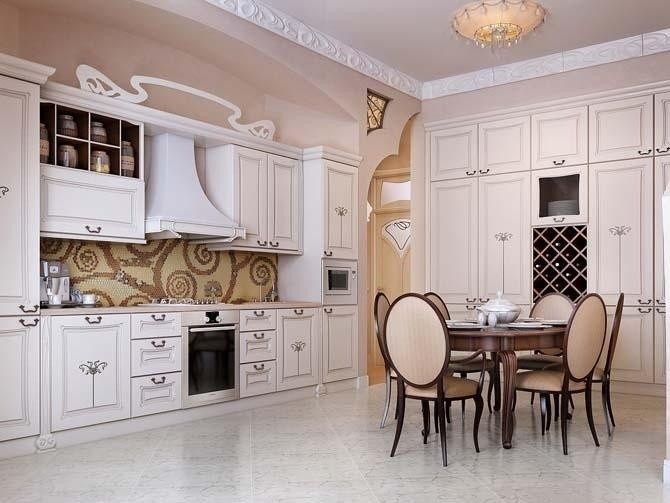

(48, 294), (62, 303)
(82, 294), (101, 303)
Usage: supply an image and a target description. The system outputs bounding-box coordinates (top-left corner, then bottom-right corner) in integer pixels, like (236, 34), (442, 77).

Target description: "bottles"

(39, 114), (135, 177)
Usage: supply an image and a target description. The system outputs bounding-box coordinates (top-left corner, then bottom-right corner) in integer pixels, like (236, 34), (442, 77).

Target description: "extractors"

(144, 133), (245, 243)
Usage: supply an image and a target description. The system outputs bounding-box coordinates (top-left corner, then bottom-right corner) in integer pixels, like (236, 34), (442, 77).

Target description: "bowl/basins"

(478, 291), (520, 323)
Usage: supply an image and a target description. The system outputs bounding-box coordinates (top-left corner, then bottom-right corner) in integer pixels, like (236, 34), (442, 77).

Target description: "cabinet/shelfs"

(130, 312), (182, 419)
(39, 307), (130, 435)
(429, 121), (478, 383)
(653, 90), (670, 385)
(530, 105), (589, 228)
(38, 95), (148, 246)
(206, 144), (306, 255)
(278, 308), (323, 393)
(240, 308), (278, 400)
(0, 51), (42, 461)
(478, 114), (531, 381)
(589, 94), (654, 385)
(278, 144), (362, 384)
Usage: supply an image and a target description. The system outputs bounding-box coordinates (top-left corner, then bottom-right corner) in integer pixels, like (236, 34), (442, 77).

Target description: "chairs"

(372, 291), (399, 431)
(515, 290), (609, 455)
(382, 292), (487, 466)
(517, 293), (577, 410)
(542, 291), (626, 436)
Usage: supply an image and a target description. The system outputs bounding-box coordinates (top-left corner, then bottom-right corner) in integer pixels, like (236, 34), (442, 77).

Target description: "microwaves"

(325, 266), (356, 295)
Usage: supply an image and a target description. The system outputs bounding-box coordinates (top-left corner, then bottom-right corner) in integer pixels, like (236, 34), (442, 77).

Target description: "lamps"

(448, 1), (550, 50)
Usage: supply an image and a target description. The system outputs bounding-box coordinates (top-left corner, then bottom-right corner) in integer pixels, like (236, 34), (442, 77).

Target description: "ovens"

(182, 311), (239, 408)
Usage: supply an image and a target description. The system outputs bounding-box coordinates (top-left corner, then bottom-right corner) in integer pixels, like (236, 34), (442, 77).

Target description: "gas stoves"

(138, 298), (233, 306)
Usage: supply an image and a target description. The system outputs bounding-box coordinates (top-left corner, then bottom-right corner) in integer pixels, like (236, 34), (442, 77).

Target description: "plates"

(76, 302), (101, 307)
(505, 320), (567, 329)
(548, 200), (578, 215)
(42, 304), (65, 308)
(447, 320), (489, 328)
